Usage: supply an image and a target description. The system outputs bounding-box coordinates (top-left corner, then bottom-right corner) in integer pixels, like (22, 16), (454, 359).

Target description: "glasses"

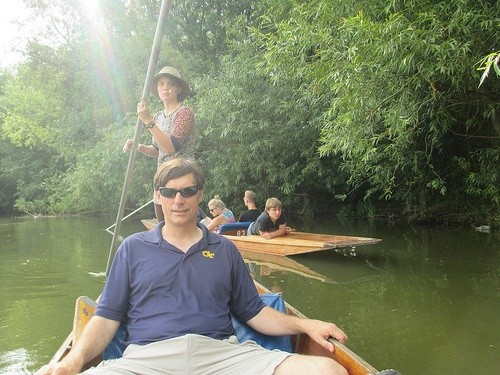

(158, 185), (199, 198)
(209, 208), (218, 212)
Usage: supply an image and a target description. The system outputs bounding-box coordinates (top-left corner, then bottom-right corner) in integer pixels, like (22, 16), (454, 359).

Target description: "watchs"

(146, 123), (156, 129)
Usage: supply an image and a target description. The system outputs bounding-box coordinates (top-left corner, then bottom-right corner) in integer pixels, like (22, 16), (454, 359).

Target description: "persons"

(123, 66), (197, 223)
(205, 195), (236, 235)
(35, 158), (349, 375)
(239, 190), (262, 222)
(247, 197), (292, 240)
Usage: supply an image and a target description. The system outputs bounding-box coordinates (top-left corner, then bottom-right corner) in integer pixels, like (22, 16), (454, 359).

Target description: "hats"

(151, 66), (191, 102)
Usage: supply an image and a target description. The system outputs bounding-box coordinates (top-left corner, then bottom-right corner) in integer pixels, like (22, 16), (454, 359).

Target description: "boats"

(30, 274), (383, 375)
(247, 252), (386, 286)
(141, 212), (383, 258)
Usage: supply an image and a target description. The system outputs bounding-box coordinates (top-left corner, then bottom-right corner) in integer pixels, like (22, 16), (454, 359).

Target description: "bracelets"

(145, 121), (153, 125)
